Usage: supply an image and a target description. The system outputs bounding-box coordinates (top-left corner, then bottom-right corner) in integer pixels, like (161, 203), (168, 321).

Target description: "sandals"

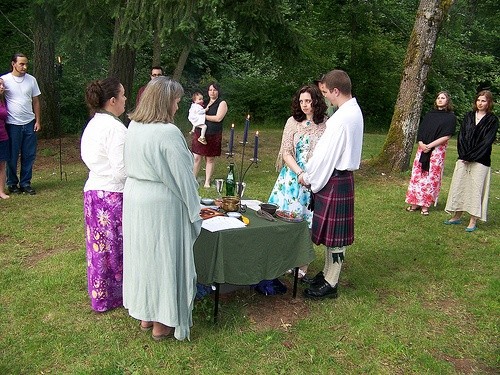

(198, 137), (207, 144)
(406, 206), (419, 211)
(189, 130), (194, 134)
(421, 209), (429, 215)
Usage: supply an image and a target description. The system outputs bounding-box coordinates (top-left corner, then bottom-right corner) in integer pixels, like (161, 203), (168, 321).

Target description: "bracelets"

(296, 170), (305, 177)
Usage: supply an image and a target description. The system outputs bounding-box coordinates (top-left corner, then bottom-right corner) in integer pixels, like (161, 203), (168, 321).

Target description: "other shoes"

(465, 226), (477, 231)
(203, 182), (211, 188)
(286, 268), (307, 279)
(445, 219), (461, 225)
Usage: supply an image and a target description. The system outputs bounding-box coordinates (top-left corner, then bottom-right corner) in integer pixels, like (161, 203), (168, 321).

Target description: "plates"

(227, 212), (242, 218)
(202, 198), (215, 204)
(274, 209), (303, 223)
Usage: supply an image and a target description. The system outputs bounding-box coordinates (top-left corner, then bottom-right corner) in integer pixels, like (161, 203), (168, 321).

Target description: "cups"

(235, 181), (246, 198)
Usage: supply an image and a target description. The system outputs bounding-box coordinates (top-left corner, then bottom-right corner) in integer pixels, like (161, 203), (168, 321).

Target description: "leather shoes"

(303, 279), (338, 299)
(302, 271), (324, 286)
(20, 186), (35, 194)
(7, 185), (21, 194)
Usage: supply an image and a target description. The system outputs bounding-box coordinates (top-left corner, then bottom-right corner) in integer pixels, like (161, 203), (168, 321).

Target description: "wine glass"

(215, 178), (225, 202)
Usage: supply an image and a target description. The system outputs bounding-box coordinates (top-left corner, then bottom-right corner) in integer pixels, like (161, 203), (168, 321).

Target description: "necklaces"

(11, 72), (25, 83)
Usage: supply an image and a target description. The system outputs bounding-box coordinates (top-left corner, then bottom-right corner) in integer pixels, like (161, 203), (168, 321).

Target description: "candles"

(56, 56), (63, 78)
(229, 123), (235, 156)
(243, 114), (251, 142)
(254, 131), (258, 161)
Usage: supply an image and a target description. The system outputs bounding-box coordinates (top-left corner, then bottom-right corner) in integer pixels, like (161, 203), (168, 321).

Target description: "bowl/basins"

(258, 203), (279, 215)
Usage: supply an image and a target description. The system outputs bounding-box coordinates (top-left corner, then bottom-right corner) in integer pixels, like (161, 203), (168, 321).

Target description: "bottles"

(225, 162), (236, 196)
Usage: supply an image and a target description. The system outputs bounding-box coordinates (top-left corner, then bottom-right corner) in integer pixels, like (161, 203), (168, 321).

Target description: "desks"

(192, 196), (311, 321)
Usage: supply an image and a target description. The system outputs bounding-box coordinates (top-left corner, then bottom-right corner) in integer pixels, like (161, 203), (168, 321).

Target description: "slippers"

(140, 325), (153, 330)
(151, 328), (175, 341)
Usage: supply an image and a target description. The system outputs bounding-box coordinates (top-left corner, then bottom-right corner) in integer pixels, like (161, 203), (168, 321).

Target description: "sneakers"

(0, 193), (10, 199)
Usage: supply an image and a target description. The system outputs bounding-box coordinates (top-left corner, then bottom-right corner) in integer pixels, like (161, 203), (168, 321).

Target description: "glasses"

(151, 74), (163, 77)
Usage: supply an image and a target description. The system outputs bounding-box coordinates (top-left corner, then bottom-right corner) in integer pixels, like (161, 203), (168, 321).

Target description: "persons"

(296, 69), (364, 299)
(188, 91), (208, 145)
(0, 52), (42, 195)
(80, 76), (129, 312)
(191, 80), (229, 188)
(313, 74), (339, 118)
(267, 83), (331, 280)
(403, 90), (457, 215)
(135, 65), (164, 108)
(0, 77), (12, 200)
(444, 89), (500, 233)
(122, 76), (203, 342)
